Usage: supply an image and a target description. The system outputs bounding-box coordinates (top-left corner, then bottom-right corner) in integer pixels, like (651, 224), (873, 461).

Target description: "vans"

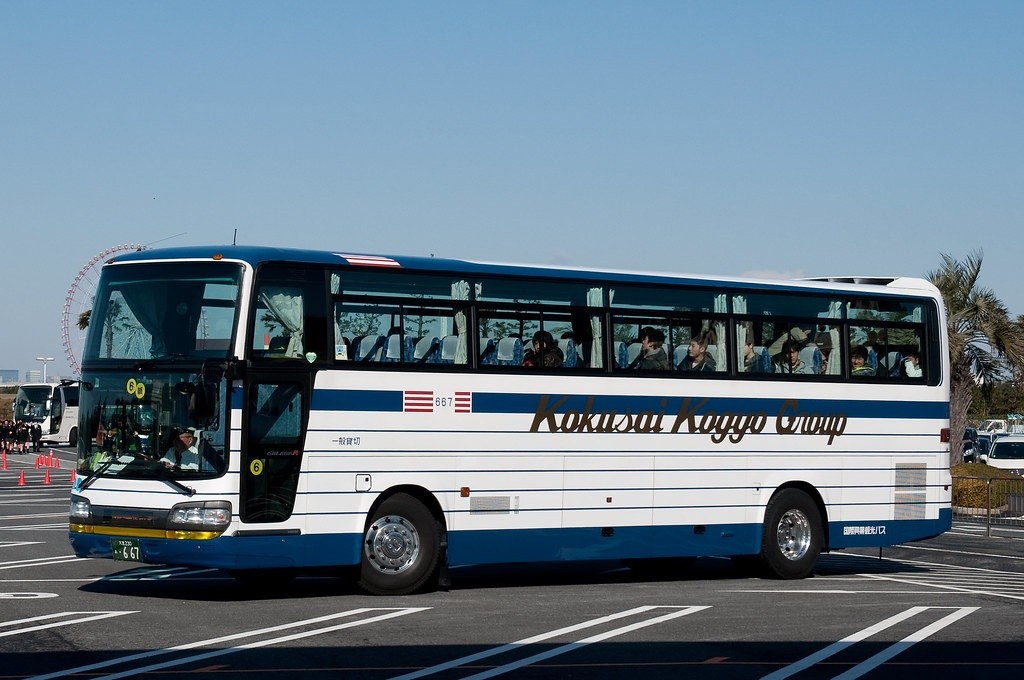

(962, 428), (980, 464)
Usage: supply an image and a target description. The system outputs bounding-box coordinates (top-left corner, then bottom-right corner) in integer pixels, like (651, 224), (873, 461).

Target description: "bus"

(68, 245), (953, 595)
(13, 381), (99, 446)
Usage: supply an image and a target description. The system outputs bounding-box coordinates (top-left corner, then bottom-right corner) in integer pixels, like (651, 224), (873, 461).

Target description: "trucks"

(977, 420), (1024, 435)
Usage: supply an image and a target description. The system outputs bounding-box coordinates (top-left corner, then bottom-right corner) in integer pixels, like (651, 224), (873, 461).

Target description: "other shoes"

(0, 448), (41, 455)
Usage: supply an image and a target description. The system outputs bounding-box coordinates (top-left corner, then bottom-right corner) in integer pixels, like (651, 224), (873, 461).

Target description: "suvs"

(986, 437), (1024, 479)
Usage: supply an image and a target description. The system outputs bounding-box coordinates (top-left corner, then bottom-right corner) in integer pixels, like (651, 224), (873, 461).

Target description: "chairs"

(261, 333), (920, 374)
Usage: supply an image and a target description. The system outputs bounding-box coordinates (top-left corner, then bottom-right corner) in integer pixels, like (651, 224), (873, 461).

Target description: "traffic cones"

(69, 468), (75, 483)
(17, 469), (26, 485)
(41, 456), (46, 466)
(54, 457), (60, 467)
(2, 450), (6, 460)
(38, 454), (42, 465)
(1, 459), (8, 470)
(34, 458), (40, 469)
(47, 455), (53, 467)
(41, 469), (53, 485)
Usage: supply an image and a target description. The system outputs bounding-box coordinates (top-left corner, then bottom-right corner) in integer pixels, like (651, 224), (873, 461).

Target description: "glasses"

(179, 435), (192, 439)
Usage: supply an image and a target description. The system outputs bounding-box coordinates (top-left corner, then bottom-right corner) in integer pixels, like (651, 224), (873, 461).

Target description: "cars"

(977, 433), (1008, 463)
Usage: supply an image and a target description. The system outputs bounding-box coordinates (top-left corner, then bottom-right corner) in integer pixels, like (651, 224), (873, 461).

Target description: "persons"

(0, 419), (42, 454)
(638, 326), (667, 370)
(521, 330), (564, 367)
(767, 323), (828, 365)
(851, 345), (874, 376)
(676, 335), (716, 371)
(508, 333), (522, 342)
(901, 352), (922, 377)
(775, 339), (815, 374)
(561, 331), (583, 367)
(159, 428), (200, 472)
(744, 334), (773, 373)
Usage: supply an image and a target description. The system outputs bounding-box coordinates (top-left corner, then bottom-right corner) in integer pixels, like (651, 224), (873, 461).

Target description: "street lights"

(36, 357), (55, 383)
